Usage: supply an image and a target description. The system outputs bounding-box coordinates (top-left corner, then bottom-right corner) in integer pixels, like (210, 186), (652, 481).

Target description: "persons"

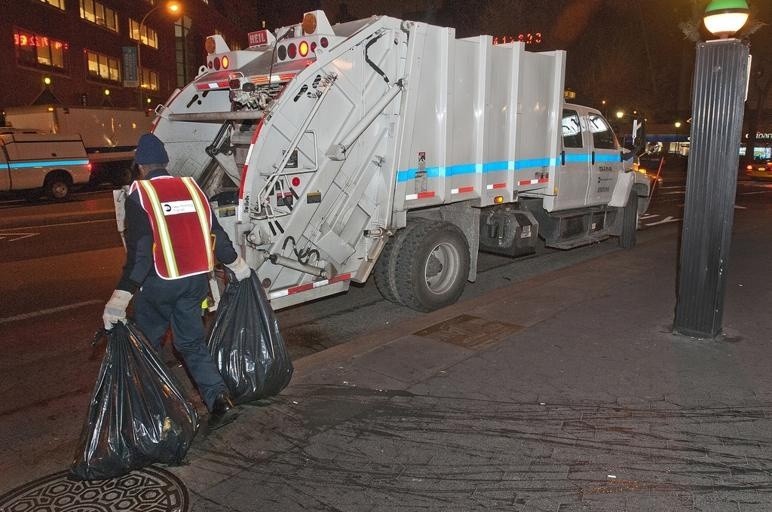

(102, 132), (251, 431)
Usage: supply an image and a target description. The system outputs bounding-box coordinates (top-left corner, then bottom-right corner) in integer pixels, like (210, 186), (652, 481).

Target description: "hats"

(134, 133), (170, 164)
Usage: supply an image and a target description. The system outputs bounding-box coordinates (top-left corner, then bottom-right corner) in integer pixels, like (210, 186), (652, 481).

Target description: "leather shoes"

(207, 390), (240, 431)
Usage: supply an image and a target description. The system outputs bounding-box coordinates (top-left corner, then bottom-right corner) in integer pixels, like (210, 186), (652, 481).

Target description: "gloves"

(223, 252), (251, 282)
(102, 290), (134, 330)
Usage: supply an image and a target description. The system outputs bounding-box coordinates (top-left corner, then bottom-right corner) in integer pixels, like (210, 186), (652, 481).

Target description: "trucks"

(2, 102), (157, 188)
(0, 129), (93, 203)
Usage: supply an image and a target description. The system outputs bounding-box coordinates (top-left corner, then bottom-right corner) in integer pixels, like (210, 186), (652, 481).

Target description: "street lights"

(674, 0), (751, 340)
(138, 0), (184, 108)
